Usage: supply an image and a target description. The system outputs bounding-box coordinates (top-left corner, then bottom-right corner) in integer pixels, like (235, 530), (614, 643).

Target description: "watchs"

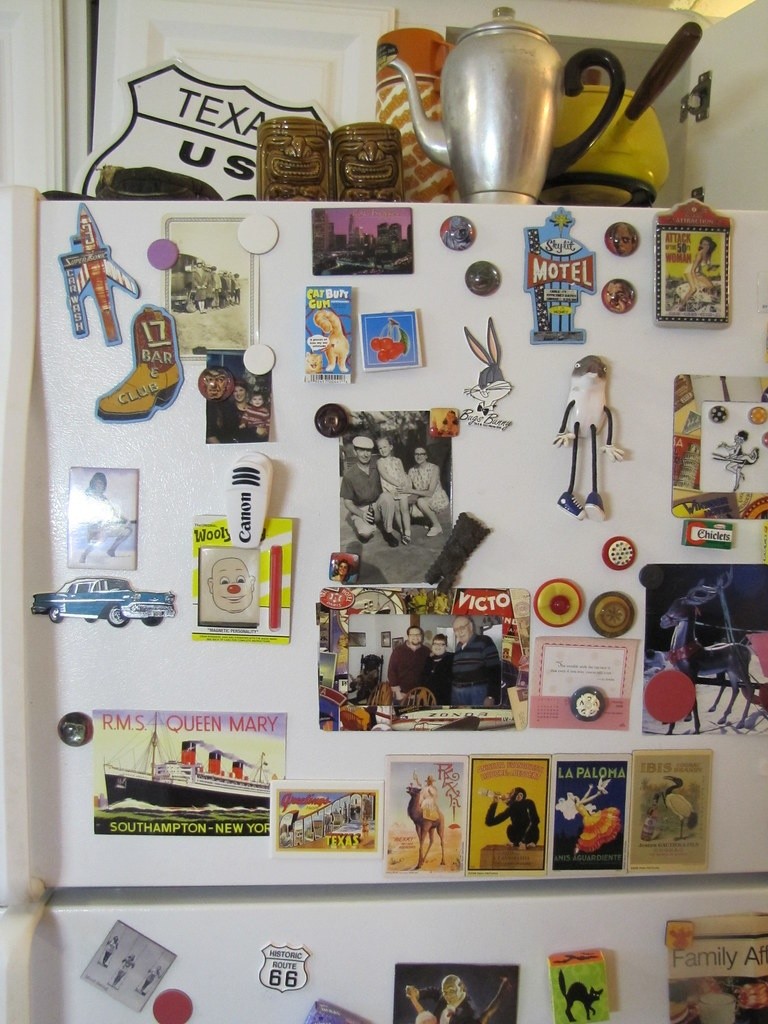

(488, 697), (493, 700)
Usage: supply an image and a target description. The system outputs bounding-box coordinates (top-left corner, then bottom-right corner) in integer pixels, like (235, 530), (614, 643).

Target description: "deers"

(659, 570), (756, 737)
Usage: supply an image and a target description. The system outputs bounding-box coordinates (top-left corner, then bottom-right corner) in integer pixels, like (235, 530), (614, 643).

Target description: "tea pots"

(386, 7), (626, 205)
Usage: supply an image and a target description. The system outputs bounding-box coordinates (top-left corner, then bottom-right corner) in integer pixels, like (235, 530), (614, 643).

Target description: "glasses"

(454, 622), (469, 633)
(414, 453), (426, 457)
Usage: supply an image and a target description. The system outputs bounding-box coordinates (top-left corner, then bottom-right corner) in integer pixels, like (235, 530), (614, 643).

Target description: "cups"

(330, 121), (404, 202)
(256, 117), (330, 202)
(377, 28), (457, 203)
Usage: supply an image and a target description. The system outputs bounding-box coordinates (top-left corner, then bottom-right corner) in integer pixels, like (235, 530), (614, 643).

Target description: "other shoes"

(384, 532), (399, 546)
(402, 534), (411, 545)
(426, 526), (442, 536)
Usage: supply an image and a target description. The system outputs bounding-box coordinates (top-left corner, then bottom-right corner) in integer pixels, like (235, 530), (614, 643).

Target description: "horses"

(406, 787), (446, 872)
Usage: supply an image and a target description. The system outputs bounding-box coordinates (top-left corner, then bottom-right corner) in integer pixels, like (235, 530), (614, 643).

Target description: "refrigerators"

(1, 188), (768, 1024)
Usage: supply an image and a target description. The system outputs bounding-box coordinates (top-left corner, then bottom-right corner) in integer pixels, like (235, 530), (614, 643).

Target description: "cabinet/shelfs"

(88, 0), (768, 209)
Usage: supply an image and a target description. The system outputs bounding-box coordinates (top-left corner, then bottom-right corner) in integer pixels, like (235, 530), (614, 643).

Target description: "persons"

(191, 258), (241, 314)
(202, 368), (232, 401)
(405, 975), (513, 1024)
(605, 279), (635, 313)
(79, 471), (132, 564)
(387, 616), (502, 708)
(485, 787), (540, 848)
(609, 223), (637, 256)
(421, 777), (440, 822)
(340, 435), (413, 547)
(677, 236), (717, 311)
(395, 447), (449, 537)
(207, 383), (270, 445)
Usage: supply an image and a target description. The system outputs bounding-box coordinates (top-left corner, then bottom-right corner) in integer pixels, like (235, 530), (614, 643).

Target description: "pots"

(536, 22), (703, 208)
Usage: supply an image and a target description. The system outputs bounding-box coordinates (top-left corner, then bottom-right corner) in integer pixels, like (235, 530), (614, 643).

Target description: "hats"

(353, 436), (374, 449)
